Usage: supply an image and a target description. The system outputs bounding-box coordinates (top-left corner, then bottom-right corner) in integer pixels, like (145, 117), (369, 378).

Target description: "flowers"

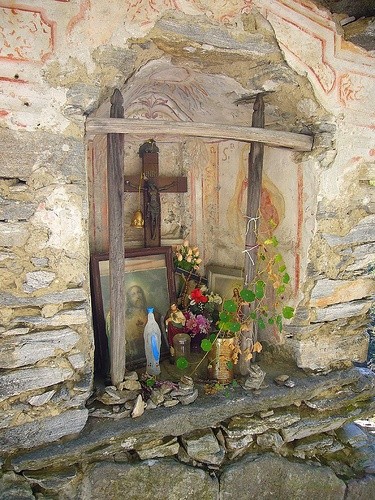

(162, 238), (221, 339)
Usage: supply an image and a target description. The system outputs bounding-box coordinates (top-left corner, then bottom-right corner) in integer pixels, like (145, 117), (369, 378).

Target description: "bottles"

(143, 307), (162, 377)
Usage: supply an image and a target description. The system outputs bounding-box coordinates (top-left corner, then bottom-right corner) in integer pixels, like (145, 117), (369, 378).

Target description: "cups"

(172, 333), (192, 377)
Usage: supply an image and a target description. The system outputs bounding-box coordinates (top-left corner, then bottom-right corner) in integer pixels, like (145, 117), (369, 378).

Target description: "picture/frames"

(90, 245), (177, 377)
